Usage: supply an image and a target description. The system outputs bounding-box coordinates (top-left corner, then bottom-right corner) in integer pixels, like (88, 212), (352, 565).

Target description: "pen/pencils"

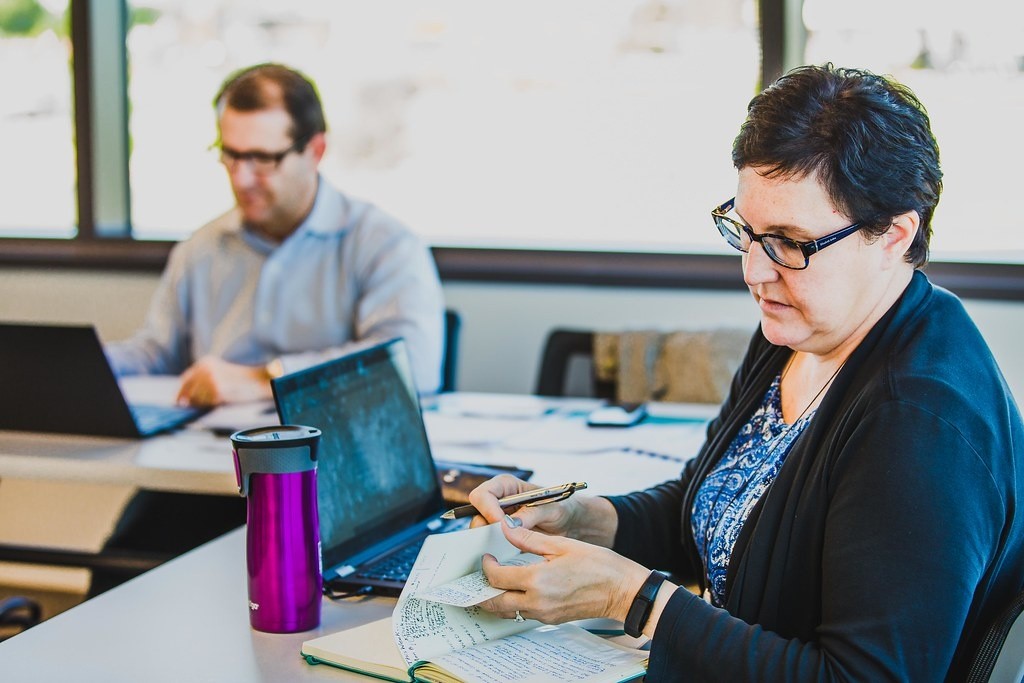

(440, 480), (589, 521)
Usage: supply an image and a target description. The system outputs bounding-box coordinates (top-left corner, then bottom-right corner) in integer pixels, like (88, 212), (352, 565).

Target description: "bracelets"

(624, 569), (668, 639)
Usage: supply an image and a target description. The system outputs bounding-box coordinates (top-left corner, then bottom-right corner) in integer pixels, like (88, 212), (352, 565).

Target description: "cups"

(231, 422), (323, 633)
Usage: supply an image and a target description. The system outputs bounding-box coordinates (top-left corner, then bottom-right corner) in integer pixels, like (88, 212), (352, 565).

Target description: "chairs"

(956, 592), (1024, 683)
(440, 306), (463, 394)
(529, 328), (753, 404)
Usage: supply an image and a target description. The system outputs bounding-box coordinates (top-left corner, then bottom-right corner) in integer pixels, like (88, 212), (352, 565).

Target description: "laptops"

(270, 336), (474, 598)
(0, 321), (217, 441)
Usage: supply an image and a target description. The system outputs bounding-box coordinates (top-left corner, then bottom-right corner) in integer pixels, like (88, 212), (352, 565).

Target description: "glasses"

(711, 196), (882, 270)
(208, 136), (305, 173)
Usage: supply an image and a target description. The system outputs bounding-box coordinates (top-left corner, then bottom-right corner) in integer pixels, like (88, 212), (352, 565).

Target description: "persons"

(468, 65), (1024, 683)
(89, 62), (445, 598)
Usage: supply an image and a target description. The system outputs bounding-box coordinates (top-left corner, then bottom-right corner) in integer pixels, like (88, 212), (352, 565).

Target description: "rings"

(513, 610), (527, 624)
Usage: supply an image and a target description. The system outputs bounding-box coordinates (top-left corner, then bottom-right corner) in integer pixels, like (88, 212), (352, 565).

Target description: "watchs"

(266, 358), (284, 377)
